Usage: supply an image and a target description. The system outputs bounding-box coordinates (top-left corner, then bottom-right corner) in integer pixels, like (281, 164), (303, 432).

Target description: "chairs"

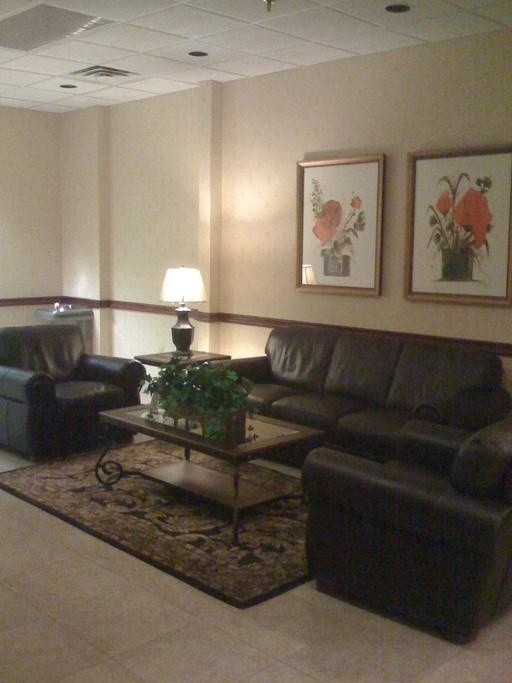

(0, 324), (147, 461)
(303, 417), (512, 645)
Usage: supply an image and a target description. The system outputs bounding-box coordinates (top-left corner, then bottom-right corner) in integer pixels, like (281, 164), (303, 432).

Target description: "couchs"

(207, 326), (511, 464)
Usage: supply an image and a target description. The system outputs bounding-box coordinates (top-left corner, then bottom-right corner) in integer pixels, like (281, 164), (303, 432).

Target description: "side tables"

(134, 352), (232, 372)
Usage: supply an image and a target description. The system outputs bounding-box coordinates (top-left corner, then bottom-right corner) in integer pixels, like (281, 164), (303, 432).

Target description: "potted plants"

(137, 352), (260, 445)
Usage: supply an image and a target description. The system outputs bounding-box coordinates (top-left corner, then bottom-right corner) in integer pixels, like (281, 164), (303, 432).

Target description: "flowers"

(426, 171), (494, 256)
(311, 179), (365, 257)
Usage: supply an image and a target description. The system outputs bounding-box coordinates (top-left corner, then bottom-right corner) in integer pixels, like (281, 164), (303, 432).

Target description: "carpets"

(0, 437), (312, 610)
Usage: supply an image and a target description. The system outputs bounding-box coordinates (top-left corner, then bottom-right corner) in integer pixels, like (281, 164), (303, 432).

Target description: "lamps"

(158, 266), (208, 357)
(301, 264), (315, 285)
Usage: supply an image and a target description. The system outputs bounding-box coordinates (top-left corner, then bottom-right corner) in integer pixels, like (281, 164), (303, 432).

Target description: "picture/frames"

(294, 152), (384, 299)
(402, 143), (512, 306)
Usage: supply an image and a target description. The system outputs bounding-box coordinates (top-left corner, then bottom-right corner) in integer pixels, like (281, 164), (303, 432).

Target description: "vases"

(439, 247), (473, 282)
(324, 253), (351, 278)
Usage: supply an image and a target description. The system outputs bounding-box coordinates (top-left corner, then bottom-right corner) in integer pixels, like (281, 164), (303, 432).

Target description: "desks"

(34, 300), (93, 341)
